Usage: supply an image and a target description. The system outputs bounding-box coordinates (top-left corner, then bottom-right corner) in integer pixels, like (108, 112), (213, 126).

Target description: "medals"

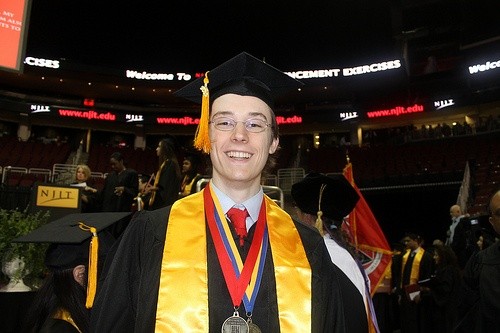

(221, 311), (261, 333)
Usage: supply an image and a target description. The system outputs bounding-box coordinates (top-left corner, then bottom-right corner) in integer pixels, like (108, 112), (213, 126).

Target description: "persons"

(14, 212), (130, 333)
(362, 114), (500, 147)
(68, 164), (101, 212)
(285, 171), (374, 290)
(101, 149), (139, 237)
(135, 137), (210, 213)
(91, 54), (348, 333)
(392, 189), (500, 333)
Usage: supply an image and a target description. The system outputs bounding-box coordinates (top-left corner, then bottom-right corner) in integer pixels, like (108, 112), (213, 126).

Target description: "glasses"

(208, 117), (275, 133)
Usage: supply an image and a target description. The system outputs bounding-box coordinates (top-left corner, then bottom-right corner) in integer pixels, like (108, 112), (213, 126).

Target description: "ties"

(226, 207), (250, 247)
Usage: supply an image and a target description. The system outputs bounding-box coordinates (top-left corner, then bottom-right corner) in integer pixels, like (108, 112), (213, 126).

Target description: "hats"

(8, 211), (133, 309)
(172, 52), (304, 155)
(291, 172), (360, 237)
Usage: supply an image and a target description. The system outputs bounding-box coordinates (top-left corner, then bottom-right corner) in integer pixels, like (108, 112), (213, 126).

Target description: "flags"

(338, 163), (393, 300)
